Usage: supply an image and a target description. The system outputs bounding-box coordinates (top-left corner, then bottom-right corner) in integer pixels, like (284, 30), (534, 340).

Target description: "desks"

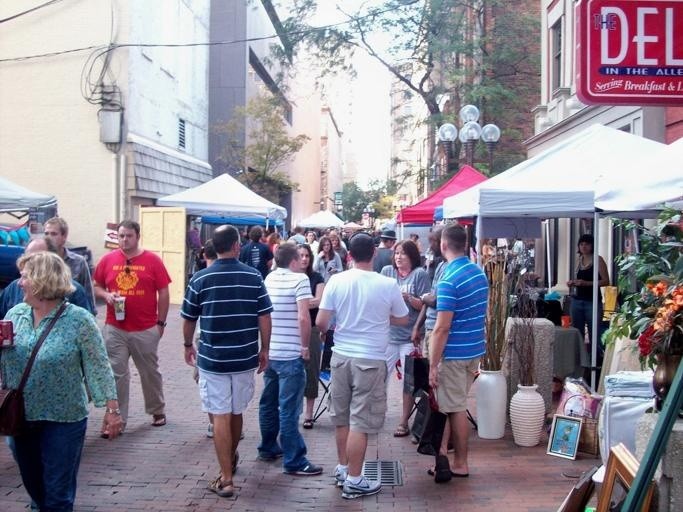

(553, 325), (591, 380)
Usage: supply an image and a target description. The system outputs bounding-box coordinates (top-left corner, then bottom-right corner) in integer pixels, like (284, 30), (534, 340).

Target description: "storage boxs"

(578, 419), (599, 455)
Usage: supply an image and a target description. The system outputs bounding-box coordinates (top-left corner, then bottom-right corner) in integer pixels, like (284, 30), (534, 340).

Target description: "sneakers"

(240, 433), (244, 440)
(447, 441), (455, 453)
(334, 462), (348, 487)
(258, 452), (283, 461)
(283, 462), (322, 474)
(341, 474), (382, 499)
(207, 423), (214, 437)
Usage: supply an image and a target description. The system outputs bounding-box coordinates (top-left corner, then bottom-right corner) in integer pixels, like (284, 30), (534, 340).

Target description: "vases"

(476, 369), (507, 440)
(652, 347), (683, 421)
(509, 384), (546, 447)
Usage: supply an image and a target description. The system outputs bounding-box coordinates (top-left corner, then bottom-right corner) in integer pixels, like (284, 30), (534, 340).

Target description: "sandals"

(219, 450), (239, 474)
(394, 424), (409, 437)
(207, 474), (233, 497)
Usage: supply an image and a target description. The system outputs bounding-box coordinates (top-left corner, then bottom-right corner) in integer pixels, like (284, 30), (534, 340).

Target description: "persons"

(408, 226), (457, 453)
(296, 245), (324, 429)
(566, 232), (609, 368)
(427, 225), (490, 484)
(378, 241), (431, 437)
(92, 220), (173, 432)
(179, 225), (273, 498)
(256, 242), (322, 474)
(189, 225), (533, 372)
(193, 239), (243, 437)
(316, 232), (410, 499)
(0, 216), (126, 511)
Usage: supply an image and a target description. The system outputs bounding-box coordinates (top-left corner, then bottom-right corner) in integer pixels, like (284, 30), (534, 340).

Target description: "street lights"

(438, 104), (500, 173)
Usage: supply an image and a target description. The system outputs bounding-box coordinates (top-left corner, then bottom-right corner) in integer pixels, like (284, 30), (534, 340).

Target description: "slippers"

(303, 419), (313, 429)
(151, 415), (166, 426)
(428, 466), (468, 477)
(102, 433), (121, 439)
(435, 455), (451, 483)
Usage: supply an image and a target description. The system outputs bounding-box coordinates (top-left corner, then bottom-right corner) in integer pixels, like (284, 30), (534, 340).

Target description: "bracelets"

(158, 319), (168, 329)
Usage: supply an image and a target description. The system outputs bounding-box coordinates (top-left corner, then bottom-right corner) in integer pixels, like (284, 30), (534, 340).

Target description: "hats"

(380, 229), (397, 240)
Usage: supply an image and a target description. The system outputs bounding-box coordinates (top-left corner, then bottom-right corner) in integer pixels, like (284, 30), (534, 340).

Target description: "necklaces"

(579, 256), (594, 270)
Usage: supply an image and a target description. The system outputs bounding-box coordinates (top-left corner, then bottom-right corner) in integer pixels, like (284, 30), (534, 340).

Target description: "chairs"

(395, 359), (479, 429)
(312, 371), (331, 422)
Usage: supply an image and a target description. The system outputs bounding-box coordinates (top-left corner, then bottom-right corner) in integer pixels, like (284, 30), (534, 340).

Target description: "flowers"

(602, 202), (683, 371)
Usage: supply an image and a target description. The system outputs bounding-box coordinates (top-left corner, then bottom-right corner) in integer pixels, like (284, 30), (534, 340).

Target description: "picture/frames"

(546, 414), (583, 460)
(595, 443), (657, 512)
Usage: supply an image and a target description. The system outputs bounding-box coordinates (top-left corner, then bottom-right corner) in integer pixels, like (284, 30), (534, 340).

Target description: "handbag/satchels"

(404, 338), (429, 397)
(0, 389), (24, 437)
(411, 384), (448, 456)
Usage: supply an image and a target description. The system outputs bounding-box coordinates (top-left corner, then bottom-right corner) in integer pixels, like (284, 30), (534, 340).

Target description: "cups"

(113, 297), (126, 320)
(561, 316), (572, 328)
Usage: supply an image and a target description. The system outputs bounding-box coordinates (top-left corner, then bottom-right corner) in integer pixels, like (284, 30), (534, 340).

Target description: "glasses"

(348, 231), (371, 241)
(296, 244), (309, 249)
(126, 257), (130, 273)
